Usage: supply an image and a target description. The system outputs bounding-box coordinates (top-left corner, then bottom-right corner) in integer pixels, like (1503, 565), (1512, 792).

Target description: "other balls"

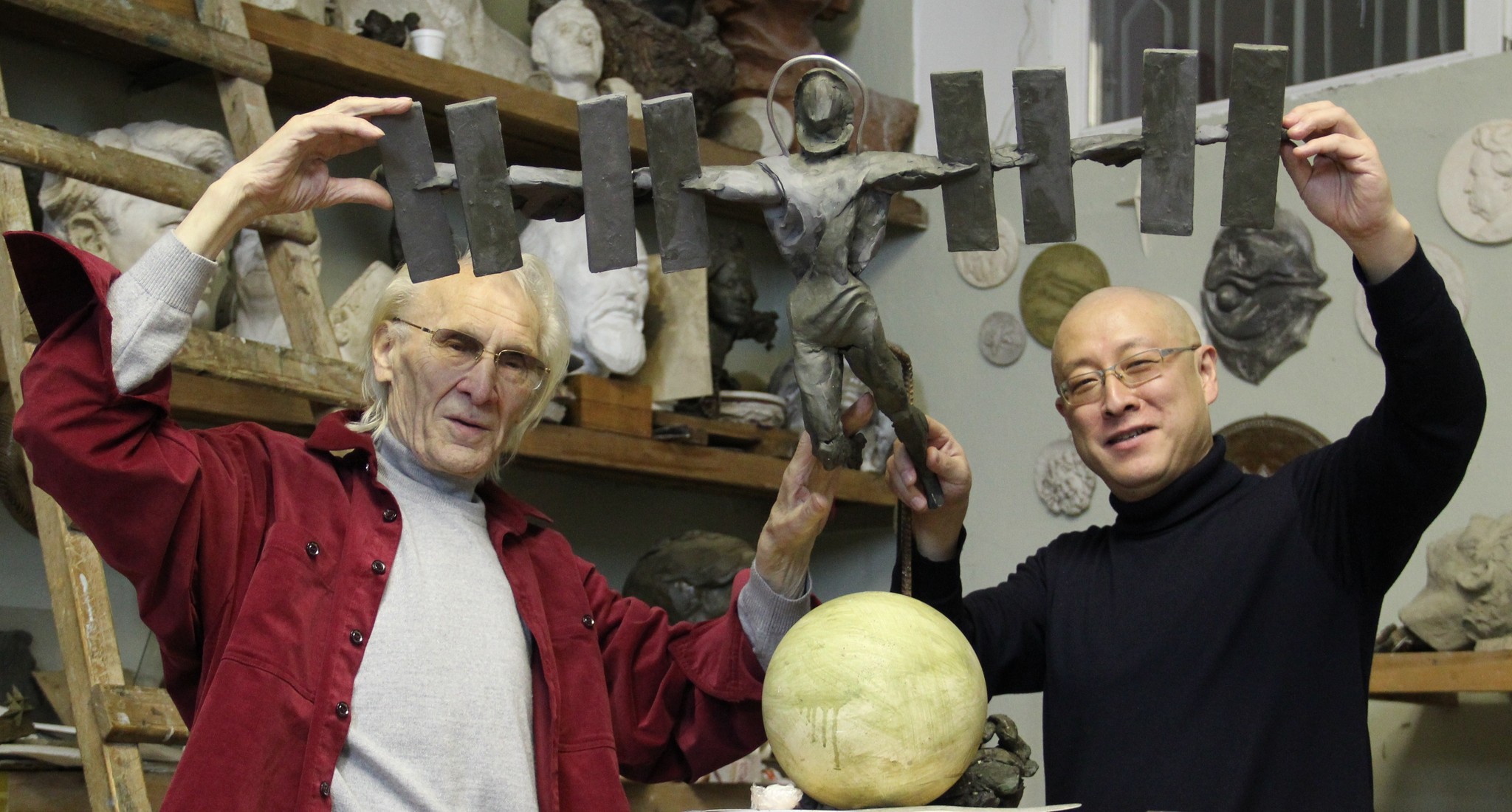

(761, 589), (989, 811)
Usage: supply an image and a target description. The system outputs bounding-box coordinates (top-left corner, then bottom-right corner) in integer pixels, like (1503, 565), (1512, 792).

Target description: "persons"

(1398, 513), (1512, 651)
(634, 54), (979, 510)
(622, 530), (756, 626)
(219, 227), (322, 349)
(531, 0), (604, 102)
(11, 96), (875, 812)
(884, 100), (1488, 812)
(673, 249), (759, 420)
(518, 213), (650, 378)
(37, 119), (233, 328)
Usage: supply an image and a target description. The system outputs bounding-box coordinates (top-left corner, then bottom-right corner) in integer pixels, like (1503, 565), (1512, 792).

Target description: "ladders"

(1, 0), (358, 810)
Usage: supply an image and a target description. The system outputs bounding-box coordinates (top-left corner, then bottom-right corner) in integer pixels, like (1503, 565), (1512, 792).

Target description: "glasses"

(1058, 341), (1202, 406)
(394, 317), (552, 393)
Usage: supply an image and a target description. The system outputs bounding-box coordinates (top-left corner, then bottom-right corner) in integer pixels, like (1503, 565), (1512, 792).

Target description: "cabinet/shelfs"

(0, 0), (930, 812)
